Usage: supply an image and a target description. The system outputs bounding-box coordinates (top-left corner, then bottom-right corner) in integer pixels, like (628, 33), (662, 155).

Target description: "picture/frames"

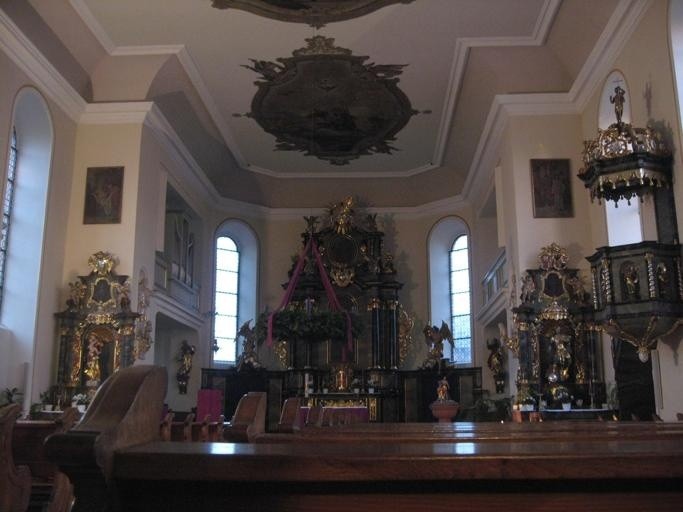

(529, 157), (574, 219)
(83, 166), (124, 226)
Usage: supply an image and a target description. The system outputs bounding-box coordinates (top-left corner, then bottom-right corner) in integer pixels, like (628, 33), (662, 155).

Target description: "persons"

(610, 86), (626, 121)
